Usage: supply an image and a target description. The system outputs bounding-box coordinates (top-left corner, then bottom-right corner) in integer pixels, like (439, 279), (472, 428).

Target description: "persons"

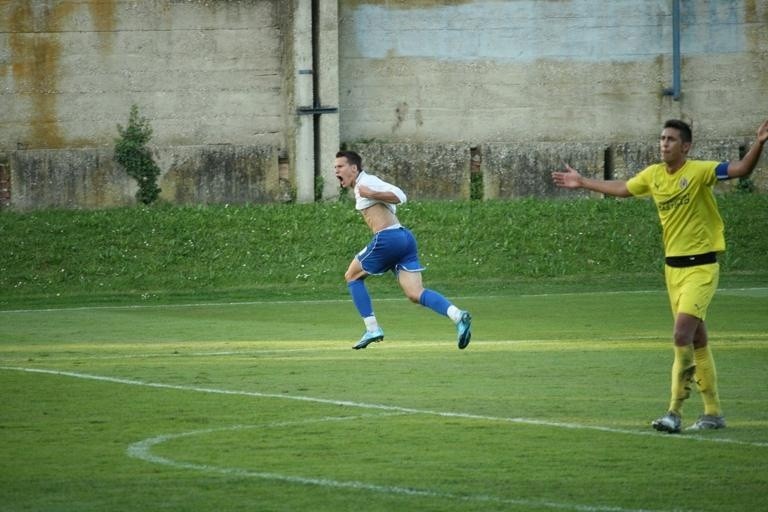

(334, 150), (472, 351)
(552, 119), (767, 433)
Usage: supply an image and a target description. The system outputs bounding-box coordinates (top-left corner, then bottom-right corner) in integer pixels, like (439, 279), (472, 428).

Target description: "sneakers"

(351, 327), (383, 349)
(685, 414), (726, 431)
(455, 311), (471, 349)
(653, 412), (681, 433)
